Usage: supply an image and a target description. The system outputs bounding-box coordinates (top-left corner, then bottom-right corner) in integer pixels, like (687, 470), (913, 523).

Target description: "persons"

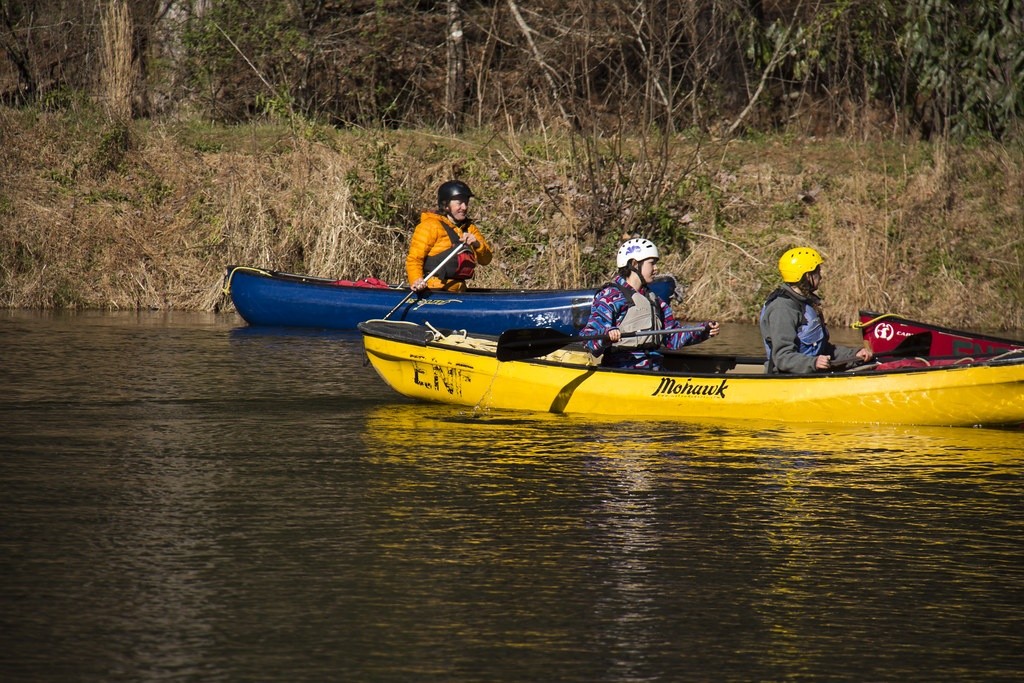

(759, 247), (873, 374)
(405, 180), (493, 292)
(579, 238), (719, 372)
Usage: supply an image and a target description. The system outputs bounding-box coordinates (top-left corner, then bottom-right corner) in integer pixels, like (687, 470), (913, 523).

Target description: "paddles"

(381, 240), (466, 321)
(496, 319), (716, 362)
(824, 331), (932, 370)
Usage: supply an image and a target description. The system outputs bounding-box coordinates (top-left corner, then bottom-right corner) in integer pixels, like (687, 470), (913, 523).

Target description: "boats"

(357, 317), (1024, 432)
(226, 263), (680, 330)
(852, 308), (1024, 362)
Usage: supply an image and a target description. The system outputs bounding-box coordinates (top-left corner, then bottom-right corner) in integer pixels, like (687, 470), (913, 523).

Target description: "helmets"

(616, 238), (660, 269)
(437, 180), (476, 207)
(778, 247), (824, 284)
